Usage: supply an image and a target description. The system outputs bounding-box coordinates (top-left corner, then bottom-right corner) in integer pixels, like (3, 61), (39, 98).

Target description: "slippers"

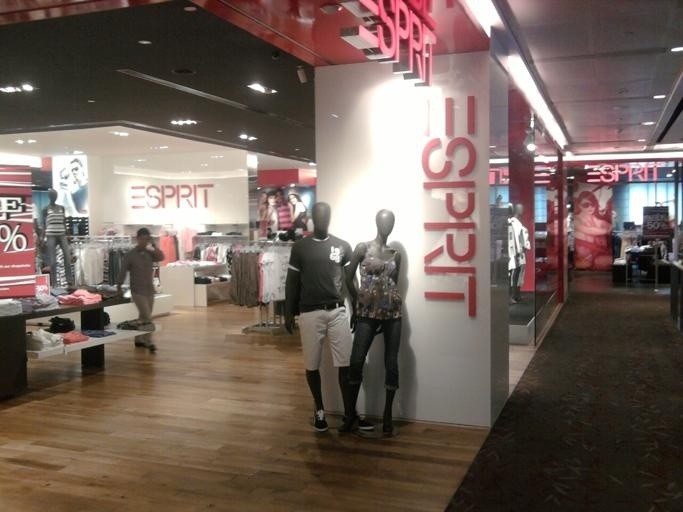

(581, 203), (594, 207)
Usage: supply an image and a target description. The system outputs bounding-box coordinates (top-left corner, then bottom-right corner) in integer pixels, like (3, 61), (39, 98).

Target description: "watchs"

(152, 242), (156, 247)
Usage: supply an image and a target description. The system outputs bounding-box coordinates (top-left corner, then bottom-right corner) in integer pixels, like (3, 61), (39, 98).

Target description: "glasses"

(71, 167), (79, 172)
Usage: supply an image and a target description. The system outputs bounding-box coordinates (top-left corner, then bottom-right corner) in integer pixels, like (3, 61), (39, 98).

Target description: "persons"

(42, 189), (73, 287)
(256, 188), (308, 230)
(575, 191), (612, 270)
(116, 227), (165, 352)
(284, 202), (376, 432)
(338, 209), (403, 437)
(508, 202), (531, 304)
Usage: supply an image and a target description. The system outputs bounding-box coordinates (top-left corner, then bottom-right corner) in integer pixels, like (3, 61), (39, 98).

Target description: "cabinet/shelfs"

(0, 280), (154, 400)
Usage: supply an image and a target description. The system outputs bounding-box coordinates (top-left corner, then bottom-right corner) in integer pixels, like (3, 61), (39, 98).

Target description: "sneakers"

(357, 415), (374, 430)
(134, 342), (158, 352)
(314, 409), (328, 431)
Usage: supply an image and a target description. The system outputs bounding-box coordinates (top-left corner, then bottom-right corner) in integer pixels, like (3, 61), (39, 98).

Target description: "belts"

(319, 303), (344, 308)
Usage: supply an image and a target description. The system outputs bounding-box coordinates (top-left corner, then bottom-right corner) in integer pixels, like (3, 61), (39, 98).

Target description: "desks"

(160, 262), (233, 308)
